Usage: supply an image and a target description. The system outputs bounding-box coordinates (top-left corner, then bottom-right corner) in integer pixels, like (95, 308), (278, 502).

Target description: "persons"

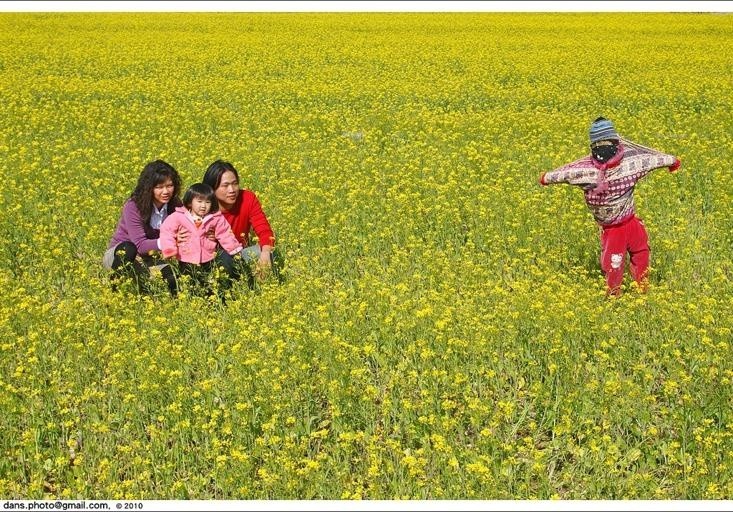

(201, 158), (277, 290)
(536, 115), (682, 298)
(158, 183), (242, 280)
(101, 159), (232, 275)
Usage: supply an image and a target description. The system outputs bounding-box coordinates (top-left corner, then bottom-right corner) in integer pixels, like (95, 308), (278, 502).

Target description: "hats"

(589, 117), (619, 143)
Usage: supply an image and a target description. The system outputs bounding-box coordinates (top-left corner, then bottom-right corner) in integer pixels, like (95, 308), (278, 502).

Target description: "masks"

(592, 144), (617, 162)
